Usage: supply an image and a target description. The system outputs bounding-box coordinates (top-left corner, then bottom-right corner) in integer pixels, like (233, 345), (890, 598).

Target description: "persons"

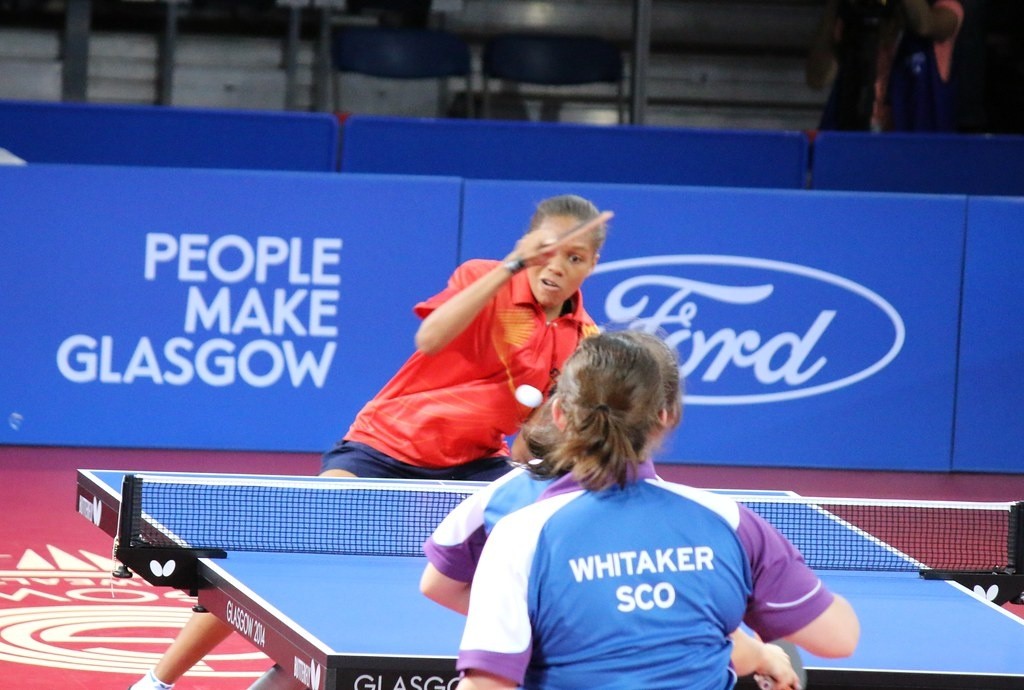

(423, 331), (861, 690)
(127, 192), (607, 690)
(808, 0), (964, 132)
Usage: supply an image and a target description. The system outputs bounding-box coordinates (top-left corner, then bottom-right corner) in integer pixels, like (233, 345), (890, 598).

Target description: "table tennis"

(516, 385), (542, 408)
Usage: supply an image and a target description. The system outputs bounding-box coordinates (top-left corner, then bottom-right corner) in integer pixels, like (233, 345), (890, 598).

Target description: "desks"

(76, 469), (1024, 690)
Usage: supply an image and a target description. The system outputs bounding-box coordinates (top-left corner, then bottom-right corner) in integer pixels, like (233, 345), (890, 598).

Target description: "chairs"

(479, 35), (626, 125)
(329, 24), (474, 120)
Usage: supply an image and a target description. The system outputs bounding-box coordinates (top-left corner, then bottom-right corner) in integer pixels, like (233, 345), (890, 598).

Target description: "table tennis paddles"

(755, 634), (807, 690)
(506, 210), (613, 273)
